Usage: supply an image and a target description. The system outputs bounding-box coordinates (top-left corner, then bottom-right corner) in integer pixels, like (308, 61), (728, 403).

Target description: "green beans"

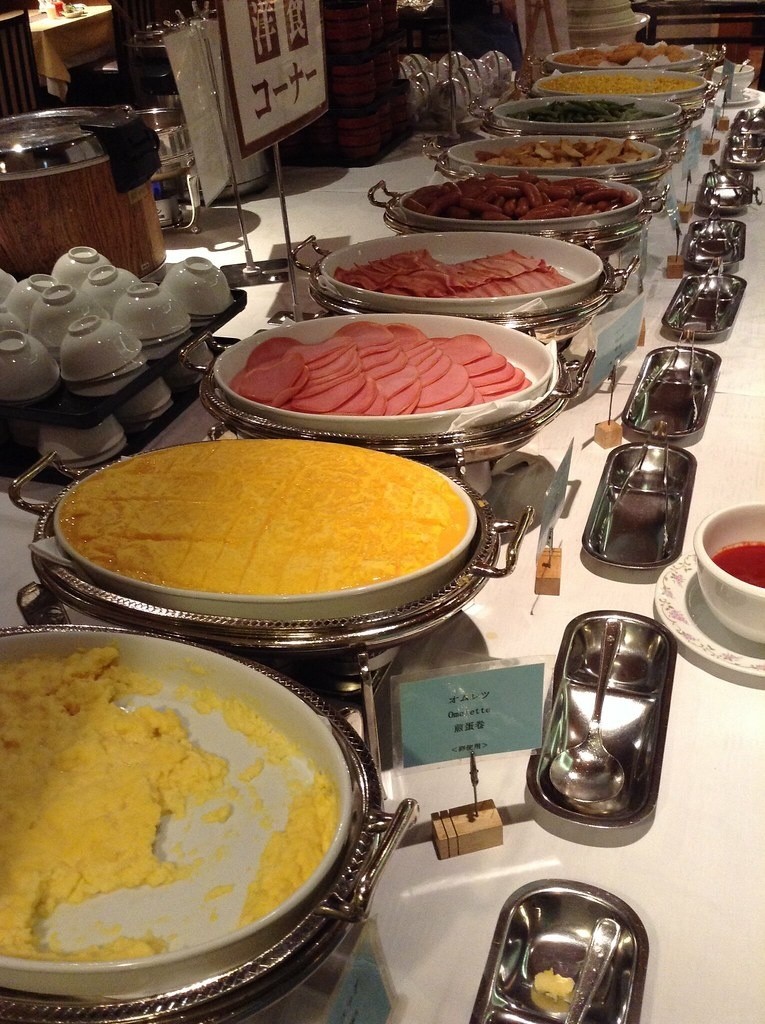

(507, 100), (649, 123)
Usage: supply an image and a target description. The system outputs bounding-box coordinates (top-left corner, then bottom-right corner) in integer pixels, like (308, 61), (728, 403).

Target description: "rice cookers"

(1, 109), (168, 283)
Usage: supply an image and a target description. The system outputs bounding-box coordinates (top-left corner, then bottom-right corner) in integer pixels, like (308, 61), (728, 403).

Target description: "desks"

(0, 5), (112, 103)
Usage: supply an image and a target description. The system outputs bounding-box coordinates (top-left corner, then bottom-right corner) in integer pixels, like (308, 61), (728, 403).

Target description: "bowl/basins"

(525, 608), (678, 828)
(680, 219), (746, 269)
(581, 439), (697, 569)
(468, 876), (651, 1024)
(0, 245), (233, 468)
(662, 273), (748, 340)
(692, 500), (765, 644)
(619, 345), (722, 445)
(695, 168), (754, 214)
(712, 63), (755, 95)
(397, 52), (513, 125)
(724, 107), (765, 169)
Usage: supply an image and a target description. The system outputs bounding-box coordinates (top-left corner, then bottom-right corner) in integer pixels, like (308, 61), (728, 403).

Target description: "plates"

(398, 175), (643, 232)
(655, 552), (765, 677)
(0, 631), (353, 1000)
(709, 89), (761, 104)
(447, 135), (662, 176)
(320, 232), (604, 313)
(212, 314), (554, 435)
(62, 9), (83, 18)
(53, 439), (477, 620)
(491, 45), (708, 132)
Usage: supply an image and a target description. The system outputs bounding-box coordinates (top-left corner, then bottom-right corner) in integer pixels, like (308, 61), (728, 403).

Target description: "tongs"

(598, 417), (671, 562)
(668, 255), (724, 328)
(706, 158), (763, 205)
(686, 204), (739, 260)
(628, 326), (697, 427)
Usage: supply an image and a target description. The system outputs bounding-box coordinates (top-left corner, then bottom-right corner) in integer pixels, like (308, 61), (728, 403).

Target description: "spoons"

(548, 619), (624, 803)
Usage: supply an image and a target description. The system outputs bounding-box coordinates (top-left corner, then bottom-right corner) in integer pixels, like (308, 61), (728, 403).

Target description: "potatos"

(475, 136), (655, 169)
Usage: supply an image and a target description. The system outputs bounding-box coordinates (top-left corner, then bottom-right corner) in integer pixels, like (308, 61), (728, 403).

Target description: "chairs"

(75, 0), (155, 103)
(0, 8), (44, 118)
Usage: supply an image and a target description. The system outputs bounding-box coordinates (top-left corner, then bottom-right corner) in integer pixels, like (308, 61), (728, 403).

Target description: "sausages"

(402, 173), (635, 221)
(229, 321), (531, 416)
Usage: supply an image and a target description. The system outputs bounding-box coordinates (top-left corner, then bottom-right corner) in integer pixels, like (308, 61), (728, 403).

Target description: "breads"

(553, 43), (690, 66)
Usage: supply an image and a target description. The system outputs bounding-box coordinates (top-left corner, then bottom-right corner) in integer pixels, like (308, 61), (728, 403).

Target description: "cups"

(38, 0), (64, 18)
